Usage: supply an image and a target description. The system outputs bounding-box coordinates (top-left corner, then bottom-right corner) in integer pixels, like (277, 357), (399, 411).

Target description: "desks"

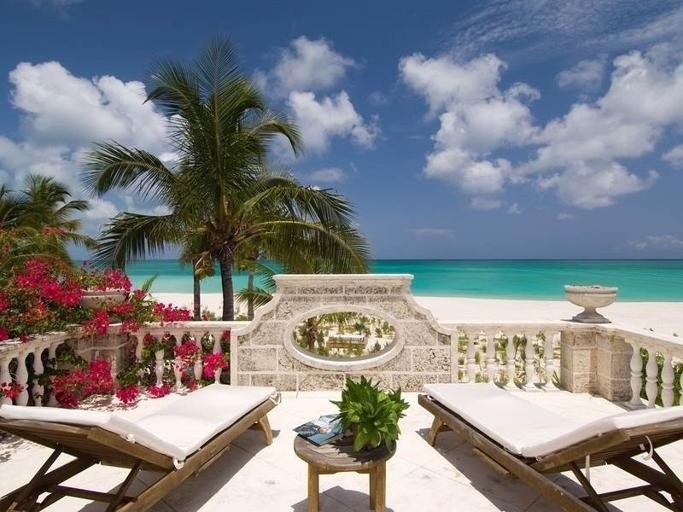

(294, 414), (397, 512)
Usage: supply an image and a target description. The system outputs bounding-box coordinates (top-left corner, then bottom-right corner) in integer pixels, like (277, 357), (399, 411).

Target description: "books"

(292, 413), (345, 446)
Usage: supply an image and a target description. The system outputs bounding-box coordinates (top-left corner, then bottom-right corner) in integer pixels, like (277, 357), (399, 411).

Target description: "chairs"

(0, 381), (284, 512)
(416, 381), (683, 512)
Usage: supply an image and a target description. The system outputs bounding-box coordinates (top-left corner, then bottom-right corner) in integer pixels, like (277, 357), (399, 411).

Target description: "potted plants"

(329, 373), (410, 453)
